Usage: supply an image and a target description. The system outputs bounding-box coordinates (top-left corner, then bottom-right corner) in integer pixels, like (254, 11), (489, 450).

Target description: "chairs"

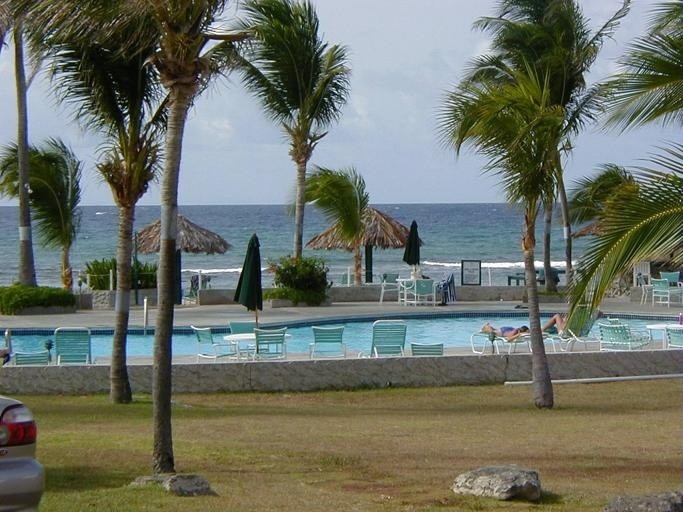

(7, 325), (92, 366)
(183, 273), (219, 304)
(472, 320), (683, 352)
(342, 271), (457, 308)
(189, 319), (444, 357)
(631, 260), (683, 308)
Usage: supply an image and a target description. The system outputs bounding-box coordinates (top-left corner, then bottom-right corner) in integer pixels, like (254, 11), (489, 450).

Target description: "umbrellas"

(231, 233), (263, 354)
(402, 219), (420, 272)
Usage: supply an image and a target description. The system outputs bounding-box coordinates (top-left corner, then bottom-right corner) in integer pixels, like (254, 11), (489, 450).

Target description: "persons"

(540, 312), (571, 340)
(481, 322), (528, 342)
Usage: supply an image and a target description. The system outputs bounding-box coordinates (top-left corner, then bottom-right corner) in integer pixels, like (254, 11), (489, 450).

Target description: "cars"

(0, 394), (48, 512)
